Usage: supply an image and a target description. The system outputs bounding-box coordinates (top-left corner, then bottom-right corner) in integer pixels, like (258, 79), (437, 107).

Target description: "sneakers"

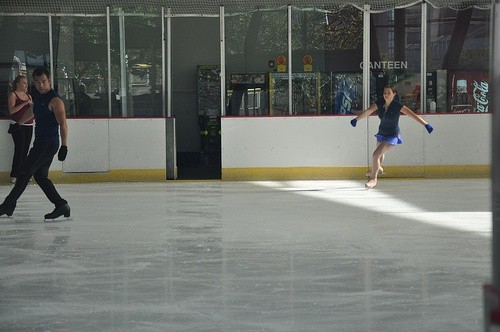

(44, 202), (70, 221)
(366, 166), (386, 177)
(0, 200), (16, 216)
(365, 178), (378, 189)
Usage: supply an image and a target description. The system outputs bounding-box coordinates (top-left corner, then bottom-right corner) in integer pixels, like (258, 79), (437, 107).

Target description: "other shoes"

(11, 177), (17, 183)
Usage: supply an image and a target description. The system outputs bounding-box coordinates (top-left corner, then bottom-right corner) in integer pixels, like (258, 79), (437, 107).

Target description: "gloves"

(58, 145), (68, 161)
(8, 123), (21, 134)
(350, 118), (357, 127)
(425, 124), (434, 134)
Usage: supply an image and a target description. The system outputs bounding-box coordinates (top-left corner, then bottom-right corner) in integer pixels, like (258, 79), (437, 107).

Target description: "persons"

(351, 84), (433, 189)
(8, 75), (34, 183)
(0, 68), (71, 219)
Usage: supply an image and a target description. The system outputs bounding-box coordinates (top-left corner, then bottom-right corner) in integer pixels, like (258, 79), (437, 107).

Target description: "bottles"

(430, 99), (436, 113)
(450, 80), (473, 113)
